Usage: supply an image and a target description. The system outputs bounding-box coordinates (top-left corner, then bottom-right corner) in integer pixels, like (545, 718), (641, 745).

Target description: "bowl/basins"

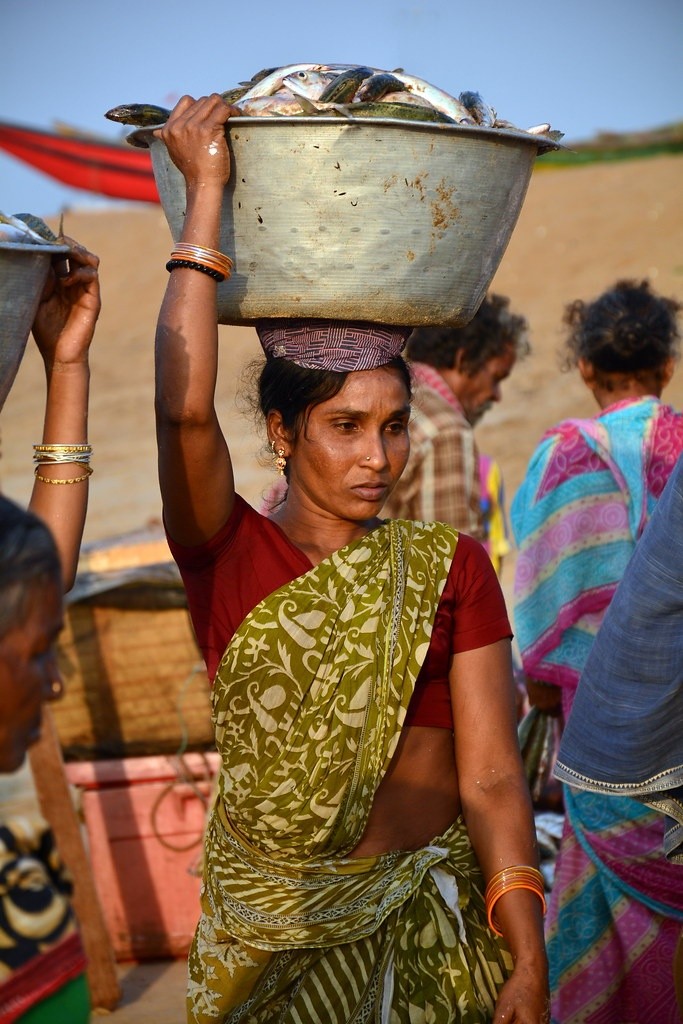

(0, 241), (70, 414)
(124, 117), (559, 328)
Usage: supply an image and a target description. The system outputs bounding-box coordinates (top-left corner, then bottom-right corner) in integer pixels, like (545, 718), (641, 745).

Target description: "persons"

(264, 289), (533, 585)
(27, 234), (102, 594)
(1, 492), (90, 1024)
(154, 92), (551, 1024)
(502, 279), (683, 814)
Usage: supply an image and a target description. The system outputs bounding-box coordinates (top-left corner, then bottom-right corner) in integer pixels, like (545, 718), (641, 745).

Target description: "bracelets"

(33, 443), (94, 484)
(165, 241), (235, 282)
(484, 864), (547, 939)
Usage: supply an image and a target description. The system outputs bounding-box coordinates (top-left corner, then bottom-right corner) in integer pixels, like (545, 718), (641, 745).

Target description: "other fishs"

(104, 60), (565, 144)
(0, 207), (65, 246)
